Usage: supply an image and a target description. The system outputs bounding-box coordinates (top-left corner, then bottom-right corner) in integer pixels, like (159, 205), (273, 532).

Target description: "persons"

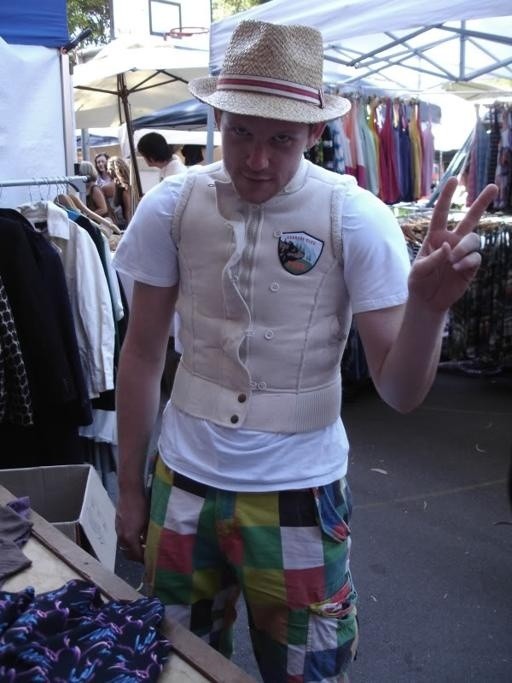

(106, 155), (132, 226)
(138, 132), (188, 183)
(78, 160), (120, 229)
(95, 152), (127, 227)
(109, 20), (500, 682)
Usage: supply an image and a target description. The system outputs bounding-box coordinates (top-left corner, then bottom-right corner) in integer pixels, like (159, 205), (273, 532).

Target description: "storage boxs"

(0, 463), (118, 574)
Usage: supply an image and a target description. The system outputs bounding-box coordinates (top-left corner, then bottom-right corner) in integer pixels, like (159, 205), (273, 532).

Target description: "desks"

(0, 485), (257, 683)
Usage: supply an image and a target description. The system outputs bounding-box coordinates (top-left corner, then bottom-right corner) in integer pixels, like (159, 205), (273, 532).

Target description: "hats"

(186, 16), (354, 126)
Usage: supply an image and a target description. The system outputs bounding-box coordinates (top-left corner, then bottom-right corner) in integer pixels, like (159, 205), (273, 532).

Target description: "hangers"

(24, 174), (120, 234)
(473, 96), (512, 110)
(323, 79), (420, 106)
(401, 219), (512, 250)
